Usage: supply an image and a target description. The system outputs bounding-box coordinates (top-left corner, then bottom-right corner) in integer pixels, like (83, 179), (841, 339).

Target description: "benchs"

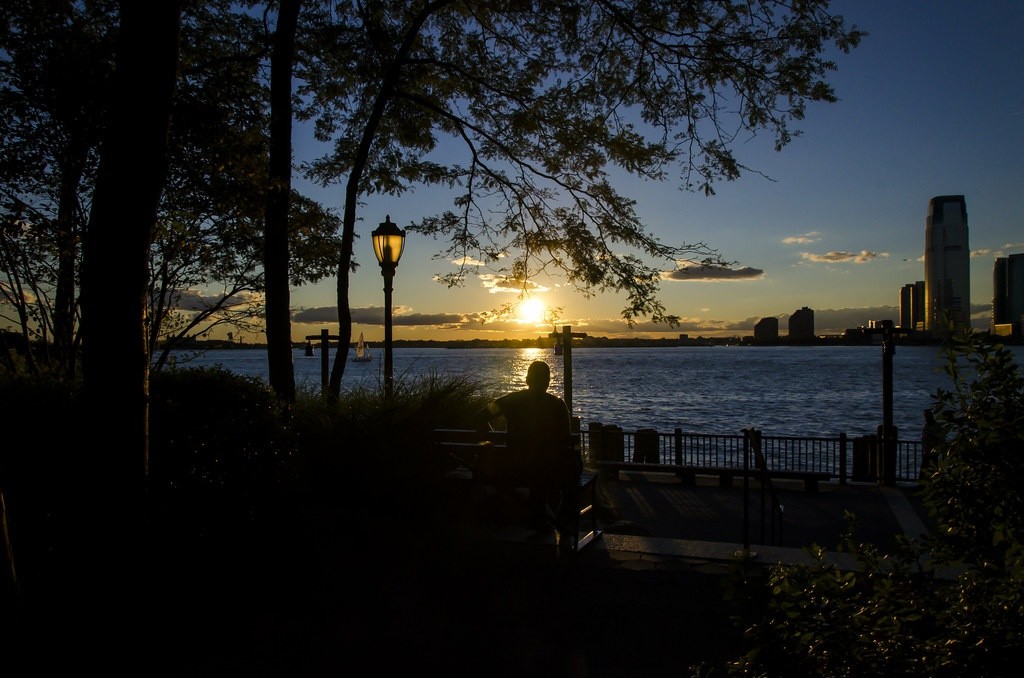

(432, 426), (604, 554)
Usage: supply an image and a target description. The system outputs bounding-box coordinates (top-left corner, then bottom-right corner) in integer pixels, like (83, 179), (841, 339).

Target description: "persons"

(475, 360), (582, 547)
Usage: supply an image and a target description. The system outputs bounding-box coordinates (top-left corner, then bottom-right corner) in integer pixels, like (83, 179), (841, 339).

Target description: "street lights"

(371, 215), (407, 405)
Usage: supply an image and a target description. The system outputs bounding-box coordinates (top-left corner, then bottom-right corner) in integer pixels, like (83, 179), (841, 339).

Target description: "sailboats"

(352, 332), (373, 362)
